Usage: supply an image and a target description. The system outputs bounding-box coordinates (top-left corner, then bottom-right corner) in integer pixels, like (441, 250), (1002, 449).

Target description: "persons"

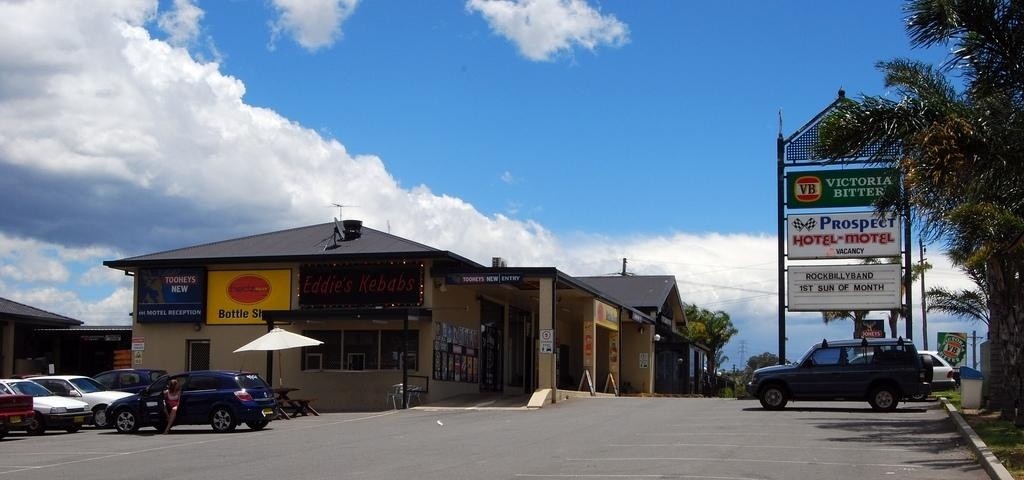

(160, 378), (181, 436)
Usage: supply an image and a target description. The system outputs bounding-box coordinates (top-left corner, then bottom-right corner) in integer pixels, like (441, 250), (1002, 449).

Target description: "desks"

(263, 387), (302, 420)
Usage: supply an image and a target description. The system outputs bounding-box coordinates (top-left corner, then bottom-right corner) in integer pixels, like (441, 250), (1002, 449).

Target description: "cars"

(0, 362), (280, 444)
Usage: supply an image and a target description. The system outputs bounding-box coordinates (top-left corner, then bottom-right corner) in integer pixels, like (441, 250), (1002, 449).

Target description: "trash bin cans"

(959, 366), (984, 409)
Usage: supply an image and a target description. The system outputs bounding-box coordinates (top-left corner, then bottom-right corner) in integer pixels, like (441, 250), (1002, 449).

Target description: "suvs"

(845, 349), (958, 404)
(743, 334), (935, 414)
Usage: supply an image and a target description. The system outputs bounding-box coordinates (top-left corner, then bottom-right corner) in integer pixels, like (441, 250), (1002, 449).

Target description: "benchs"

(286, 398), (321, 419)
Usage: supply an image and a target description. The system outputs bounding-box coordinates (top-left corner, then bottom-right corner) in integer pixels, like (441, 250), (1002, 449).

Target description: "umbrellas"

(230, 324), (324, 387)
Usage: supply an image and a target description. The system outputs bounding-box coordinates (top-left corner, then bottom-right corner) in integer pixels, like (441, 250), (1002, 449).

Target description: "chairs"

(386, 383), (424, 410)
